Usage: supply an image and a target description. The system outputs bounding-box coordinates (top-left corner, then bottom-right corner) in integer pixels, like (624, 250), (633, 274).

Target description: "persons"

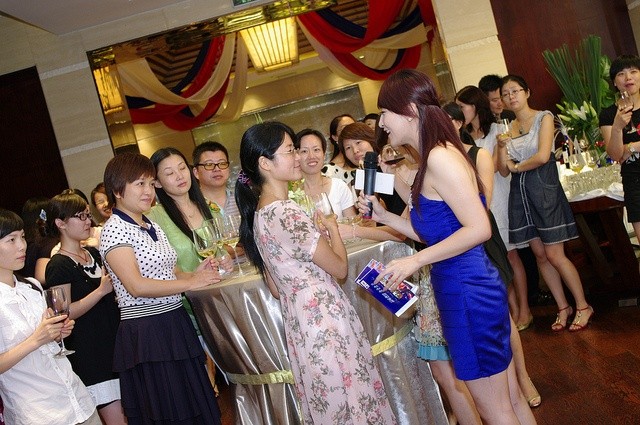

(92, 184), (111, 216)
(235, 121), (398, 424)
(0, 209), (101, 424)
(451, 86), (534, 332)
(322, 113), (354, 189)
(497, 77), (594, 333)
(99, 154), (221, 425)
(292, 128), (357, 222)
(341, 123), (379, 168)
(357, 71), (536, 425)
(475, 72), (508, 120)
(319, 199), (484, 425)
(145, 147), (225, 394)
(598, 52), (640, 242)
(438, 98), (541, 406)
(36, 194), (127, 424)
(192, 140), (241, 243)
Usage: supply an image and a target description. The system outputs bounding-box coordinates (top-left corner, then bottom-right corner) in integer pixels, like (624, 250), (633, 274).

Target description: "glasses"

(503, 89), (524, 98)
(198, 161), (229, 170)
(71, 212), (92, 220)
(272, 148), (300, 156)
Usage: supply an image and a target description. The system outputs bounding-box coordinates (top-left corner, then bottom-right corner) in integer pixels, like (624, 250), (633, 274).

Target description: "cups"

(567, 164), (626, 198)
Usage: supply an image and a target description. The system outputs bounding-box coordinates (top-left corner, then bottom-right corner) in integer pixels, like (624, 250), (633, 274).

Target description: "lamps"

(218, 0), (334, 75)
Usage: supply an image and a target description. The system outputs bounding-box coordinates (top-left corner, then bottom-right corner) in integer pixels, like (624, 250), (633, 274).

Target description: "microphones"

(363, 152), (377, 220)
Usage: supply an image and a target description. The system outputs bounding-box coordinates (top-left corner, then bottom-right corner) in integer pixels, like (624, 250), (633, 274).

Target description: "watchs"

(628, 142), (637, 154)
(515, 163), (520, 174)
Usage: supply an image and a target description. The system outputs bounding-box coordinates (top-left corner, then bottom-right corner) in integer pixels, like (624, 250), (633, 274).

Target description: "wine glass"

(190, 226), (223, 281)
(613, 88), (633, 132)
(497, 119), (516, 160)
(569, 154), (589, 196)
(586, 149), (599, 170)
(306, 191), (338, 247)
(202, 218), (237, 277)
(44, 285), (76, 359)
(381, 145), (404, 182)
(214, 215), (251, 278)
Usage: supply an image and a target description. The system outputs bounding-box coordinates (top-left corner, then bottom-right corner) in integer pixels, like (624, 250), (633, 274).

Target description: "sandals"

(551, 305), (574, 331)
(528, 377), (541, 407)
(516, 315), (534, 331)
(569, 304), (594, 331)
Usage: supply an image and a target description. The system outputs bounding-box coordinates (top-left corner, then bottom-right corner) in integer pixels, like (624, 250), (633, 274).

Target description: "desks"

(181, 225), (405, 425)
(557, 158), (640, 311)
